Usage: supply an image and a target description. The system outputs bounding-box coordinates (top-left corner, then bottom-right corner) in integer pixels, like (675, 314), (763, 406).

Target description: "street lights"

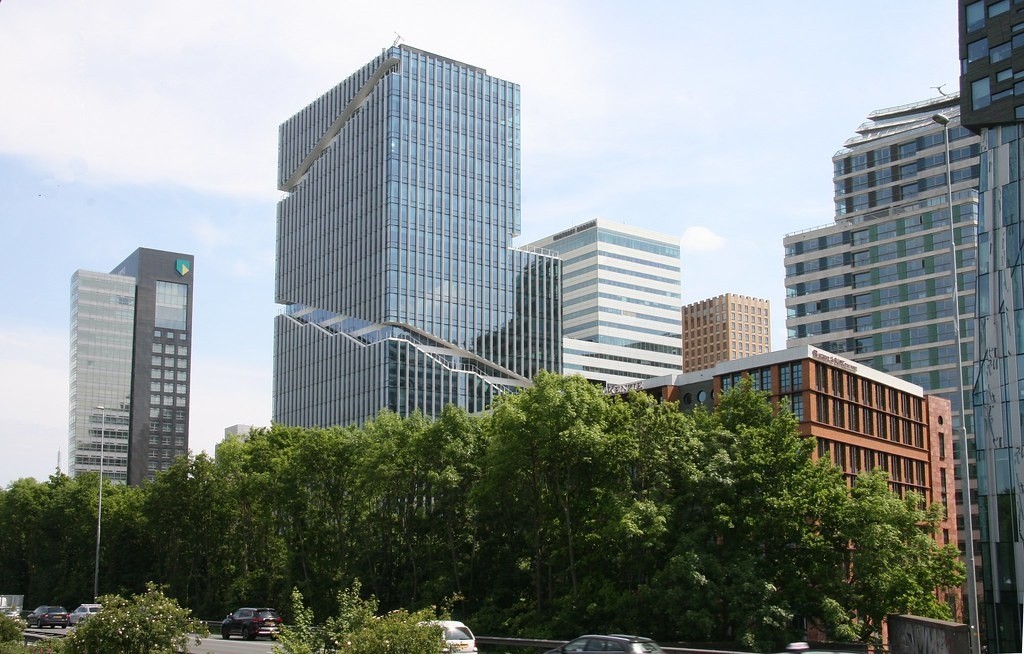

(95, 406), (105, 601)
(933, 111), (981, 654)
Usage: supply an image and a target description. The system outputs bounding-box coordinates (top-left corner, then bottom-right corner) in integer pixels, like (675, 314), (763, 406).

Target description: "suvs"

(221, 608), (283, 641)
(543, 634), (665, 654)
(418, 622), (478, 654)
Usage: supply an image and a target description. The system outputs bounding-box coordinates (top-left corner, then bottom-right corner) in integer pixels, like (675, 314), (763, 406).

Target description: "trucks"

(0, 595), (24, 618)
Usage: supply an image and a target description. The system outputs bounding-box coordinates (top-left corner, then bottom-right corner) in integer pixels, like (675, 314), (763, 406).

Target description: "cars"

(69, 606), (102, 626)
(26, 606), (68, 629)
